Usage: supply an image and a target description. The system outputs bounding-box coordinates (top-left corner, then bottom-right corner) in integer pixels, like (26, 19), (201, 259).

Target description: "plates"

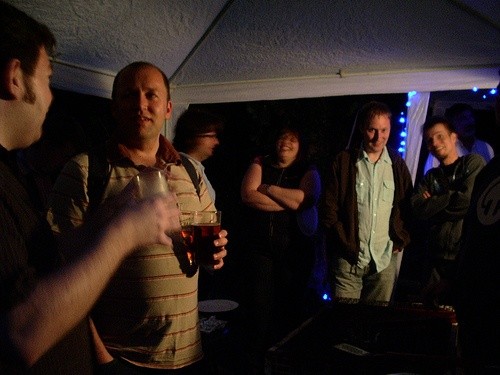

(198, 300), (238, 313)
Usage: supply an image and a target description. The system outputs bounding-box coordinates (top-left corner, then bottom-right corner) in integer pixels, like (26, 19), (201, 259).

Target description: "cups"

(136, 173), (168, 199)
(192, 211), (221, 264)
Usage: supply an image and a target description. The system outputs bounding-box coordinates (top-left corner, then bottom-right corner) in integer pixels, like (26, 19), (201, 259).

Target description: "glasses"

(196, 134), (218, 138)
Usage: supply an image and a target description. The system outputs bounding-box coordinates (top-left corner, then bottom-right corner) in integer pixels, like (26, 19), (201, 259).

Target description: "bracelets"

(264, 184), (271, 192)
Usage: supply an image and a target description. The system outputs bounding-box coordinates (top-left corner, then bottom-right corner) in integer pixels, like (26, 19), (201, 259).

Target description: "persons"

(35, 62), (500, 375)
(0, 0), (182, 375)
